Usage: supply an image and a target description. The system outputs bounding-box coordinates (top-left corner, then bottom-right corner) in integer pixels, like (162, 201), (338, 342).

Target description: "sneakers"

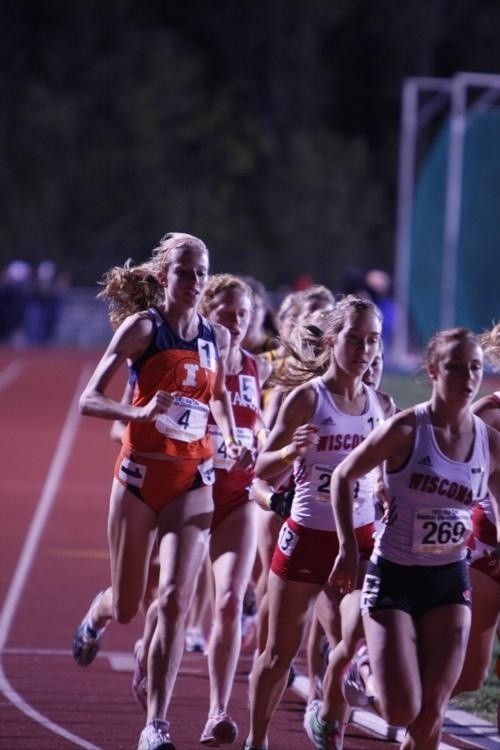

(244, 587), (258, 615)
(71, 590), (111, 668)
(242, 619), (254, 650)
(199, 713), (238, 746)
(346, 641), (377, 707)
(303, 700), (342, 749)
(133, 639), (149, 711)
(136, 720), (175, 750)
(185, 629), (206, 654)
(242, 736), (268, 750)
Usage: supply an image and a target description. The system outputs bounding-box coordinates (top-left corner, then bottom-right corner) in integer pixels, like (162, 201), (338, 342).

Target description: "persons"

(1, 260), (67, 349)
(72, 233), (254, 750)
(110, 268), (500, 750)
